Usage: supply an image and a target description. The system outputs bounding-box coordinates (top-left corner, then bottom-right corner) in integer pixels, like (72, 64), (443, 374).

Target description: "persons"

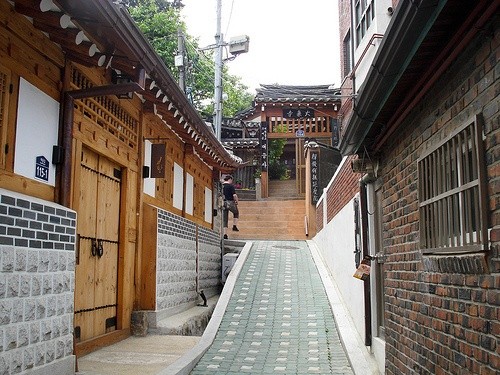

(222, 176), (240, 239)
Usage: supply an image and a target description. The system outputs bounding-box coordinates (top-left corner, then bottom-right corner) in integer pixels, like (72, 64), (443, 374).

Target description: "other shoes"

(233, 225), (239, 231)
(224, 234), (228, 239)
